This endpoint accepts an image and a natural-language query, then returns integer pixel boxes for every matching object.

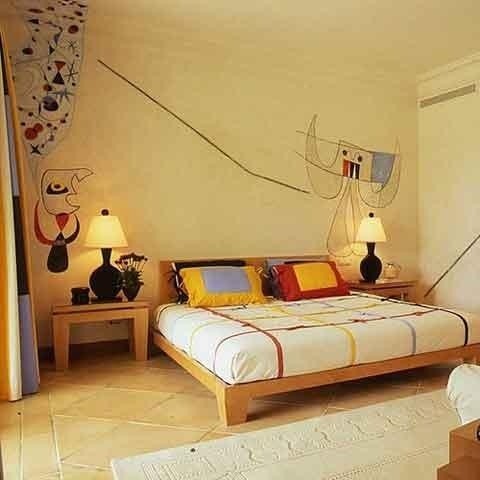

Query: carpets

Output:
[107,388,461,480]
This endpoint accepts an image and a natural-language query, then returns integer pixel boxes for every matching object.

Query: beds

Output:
[152,251,480,427]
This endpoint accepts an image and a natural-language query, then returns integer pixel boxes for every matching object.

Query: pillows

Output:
[179,266,270,309]
[267,261,352,303]
[265,258,313,300]
[169,258,247,305]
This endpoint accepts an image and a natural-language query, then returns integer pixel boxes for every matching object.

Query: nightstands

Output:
[350,277,419,305]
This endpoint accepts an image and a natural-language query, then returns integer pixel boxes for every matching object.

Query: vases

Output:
[121,283,141,302]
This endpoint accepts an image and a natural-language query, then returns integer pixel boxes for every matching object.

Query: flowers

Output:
[113,251,150,287]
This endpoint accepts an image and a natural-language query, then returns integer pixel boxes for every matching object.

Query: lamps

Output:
[354,210,388,284]
[84,207,129,300]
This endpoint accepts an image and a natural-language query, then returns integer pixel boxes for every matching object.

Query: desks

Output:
[49,294,154,373]
